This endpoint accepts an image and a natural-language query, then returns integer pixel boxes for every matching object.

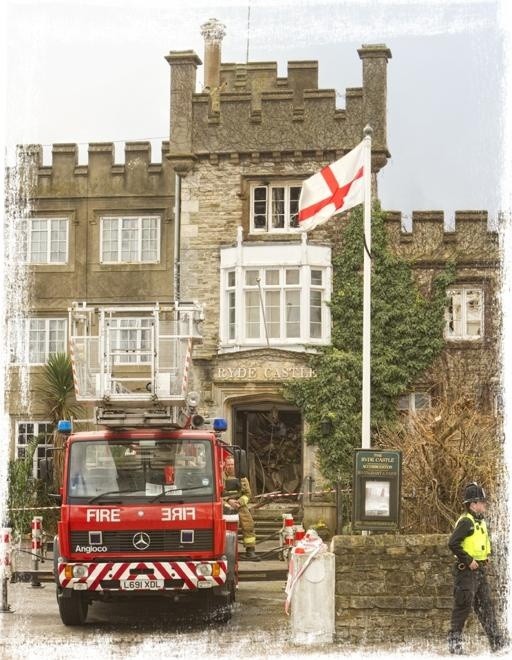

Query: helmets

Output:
[461,484,487,505]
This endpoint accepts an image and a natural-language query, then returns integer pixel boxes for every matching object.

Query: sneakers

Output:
[446,630,511,655]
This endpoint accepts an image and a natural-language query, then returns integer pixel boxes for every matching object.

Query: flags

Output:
[297,137,364,233]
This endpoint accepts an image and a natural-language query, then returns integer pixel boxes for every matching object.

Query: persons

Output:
[222,455,261,563]
[446,481,511,656]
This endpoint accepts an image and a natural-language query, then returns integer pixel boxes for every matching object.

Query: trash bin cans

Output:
[291,552,335,643]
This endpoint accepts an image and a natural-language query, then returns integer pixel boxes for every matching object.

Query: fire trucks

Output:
[44,412,248,626]
